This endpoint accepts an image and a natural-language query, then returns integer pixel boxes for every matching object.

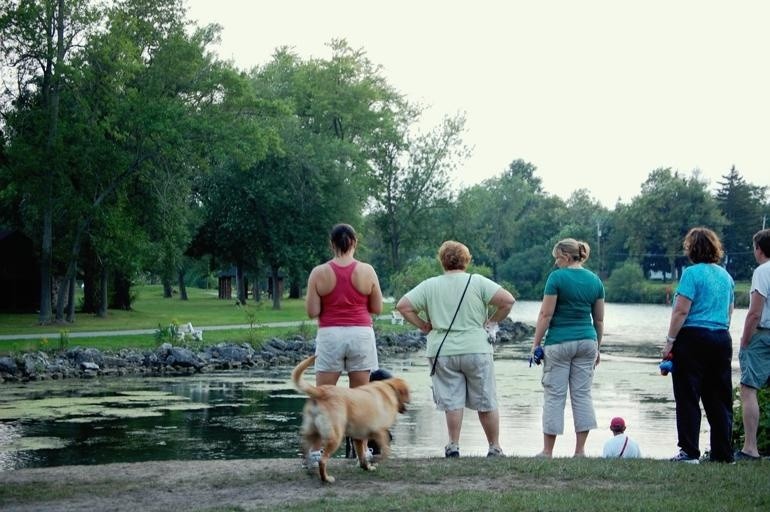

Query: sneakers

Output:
[670,450,700,465]
[701,455,736,465]
[487,446,504,457]
[356,448,374,468]
[301,449,321,468]
[444,442,460,457]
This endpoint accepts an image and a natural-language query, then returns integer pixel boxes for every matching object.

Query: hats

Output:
[610,417,625,430]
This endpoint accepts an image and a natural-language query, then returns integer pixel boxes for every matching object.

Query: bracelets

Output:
[665,334,677,346]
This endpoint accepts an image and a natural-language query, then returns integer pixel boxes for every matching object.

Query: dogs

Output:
[290,354,411,484]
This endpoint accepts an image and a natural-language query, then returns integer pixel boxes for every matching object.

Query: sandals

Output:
[734,451,761,464]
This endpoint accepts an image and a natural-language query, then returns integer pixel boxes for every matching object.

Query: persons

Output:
[529,237,599,457]
[603,417,640,455]
[732,229,769,461]
[303,223,383,470]
[658,226,735,465]
[394,239,515,458]
